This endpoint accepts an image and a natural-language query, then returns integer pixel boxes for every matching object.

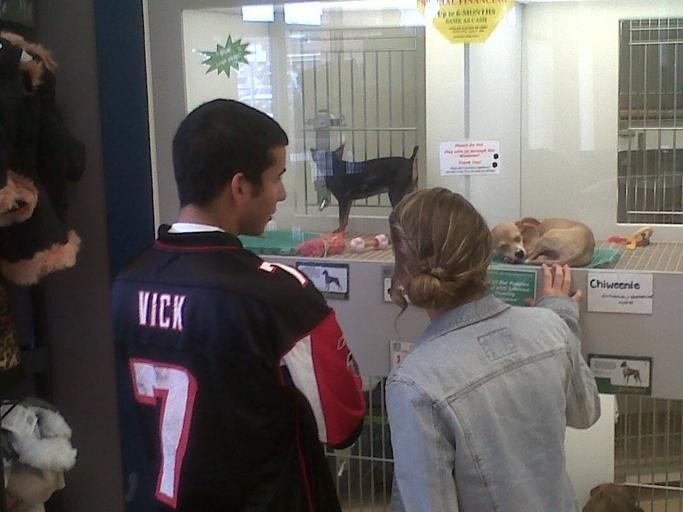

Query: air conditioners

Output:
[620,361,643,384]
[490,217,596,268]
[310,142,419,234]
[322,270,341,292]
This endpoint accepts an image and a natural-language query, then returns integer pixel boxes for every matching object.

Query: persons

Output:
[109,99,365,511]
[382,187,599,511]
